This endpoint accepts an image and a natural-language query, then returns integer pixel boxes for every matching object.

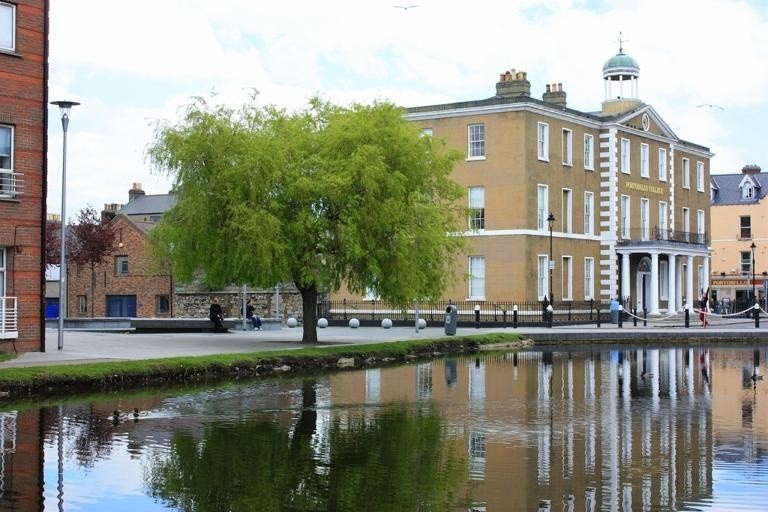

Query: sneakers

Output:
[254,326,264,330]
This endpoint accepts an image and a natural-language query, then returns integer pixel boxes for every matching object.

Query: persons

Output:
[241,299,264,331]
[209,296,224,327]
[700,284,710,326]
[700,351,713,387]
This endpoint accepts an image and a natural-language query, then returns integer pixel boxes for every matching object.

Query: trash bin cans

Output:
[445,305,456,335]
[610,300,619,323]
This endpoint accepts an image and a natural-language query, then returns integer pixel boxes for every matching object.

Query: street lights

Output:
[545,212,554,305]
[50,99,80,351]
[749,242,756,319]
